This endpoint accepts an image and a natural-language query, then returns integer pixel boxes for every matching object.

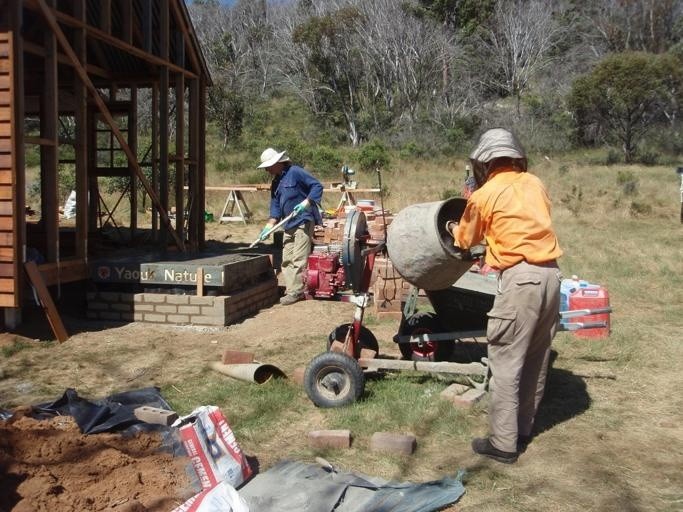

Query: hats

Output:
[256,148,287,169]
[468,127,524,163]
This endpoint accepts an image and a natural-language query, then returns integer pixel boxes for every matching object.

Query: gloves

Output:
[293,199,311,214]
[259,223,274,241]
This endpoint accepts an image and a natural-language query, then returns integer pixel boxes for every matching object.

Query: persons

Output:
[449,127,564,462]
[257,147,324,305]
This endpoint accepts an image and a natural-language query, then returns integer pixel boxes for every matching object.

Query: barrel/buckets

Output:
[569,287,613,340]
[569,282,601,295]
[560,274,586,330]
[385,196,480,291]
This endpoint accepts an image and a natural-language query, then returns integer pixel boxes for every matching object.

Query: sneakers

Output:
[471,437,518,464]
[280,292,306,304]
[518,435,529,453]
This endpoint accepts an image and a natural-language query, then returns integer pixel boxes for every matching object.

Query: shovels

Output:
[227,211,298,252]
